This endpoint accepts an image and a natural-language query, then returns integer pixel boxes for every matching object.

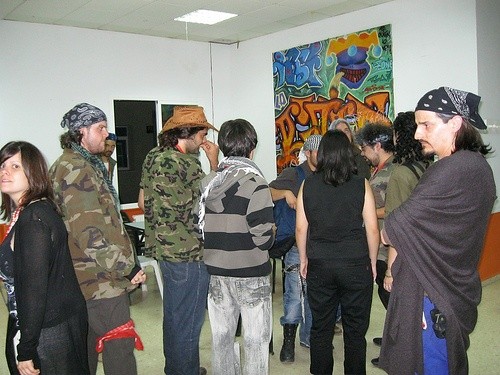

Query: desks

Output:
[125,222,146,255]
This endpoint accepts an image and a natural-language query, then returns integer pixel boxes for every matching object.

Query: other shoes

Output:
[164,366,207,375]
[371,358,380,366]
[373,338,382,345]
[334,326,342,333]
[300,342,334,350]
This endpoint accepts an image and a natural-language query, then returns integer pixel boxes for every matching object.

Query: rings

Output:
[135,278,139,282]
[141,272,145,276]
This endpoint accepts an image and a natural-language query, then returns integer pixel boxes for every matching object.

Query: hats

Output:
[160,104,220,133]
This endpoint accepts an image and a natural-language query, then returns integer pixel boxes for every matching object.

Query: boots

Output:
[280,324,299,363]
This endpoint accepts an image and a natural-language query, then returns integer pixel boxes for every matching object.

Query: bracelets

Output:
[385,274,392,277]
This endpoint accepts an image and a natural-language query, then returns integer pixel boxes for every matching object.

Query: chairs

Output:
[137,255,164,300]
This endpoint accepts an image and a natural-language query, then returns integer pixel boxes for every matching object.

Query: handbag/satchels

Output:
[268,235,295,258]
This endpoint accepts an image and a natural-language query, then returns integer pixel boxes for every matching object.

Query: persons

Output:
[381,87,498,375]
[295,129,380,375]
[0,142,89,375]
[138,106,220,375]
[356,112,435,369]
[268,135,323,364]
[47,103,146,375]
[203,119,277,374]
[328,119,361,335]
